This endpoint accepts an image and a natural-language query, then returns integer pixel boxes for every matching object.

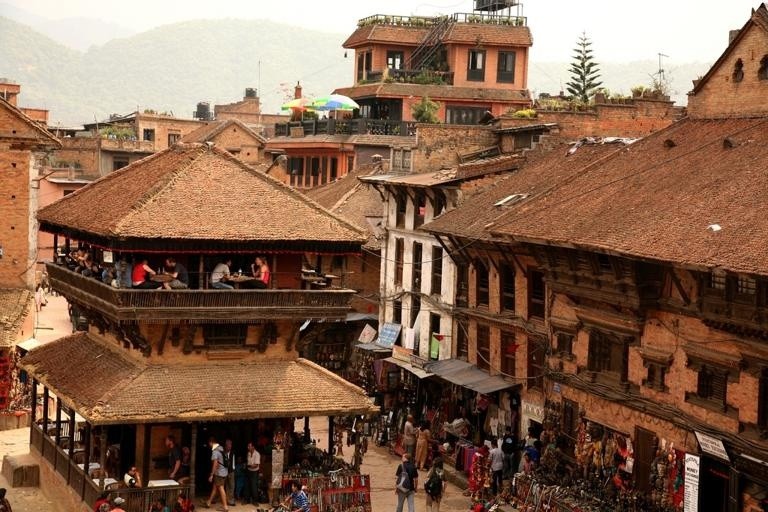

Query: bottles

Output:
[238,267,242,277]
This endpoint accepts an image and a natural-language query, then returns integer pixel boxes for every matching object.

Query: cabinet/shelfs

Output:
[513,473,599,511]
[315,343,346,376]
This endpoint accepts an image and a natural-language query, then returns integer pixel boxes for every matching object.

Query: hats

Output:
[114,497,126,504]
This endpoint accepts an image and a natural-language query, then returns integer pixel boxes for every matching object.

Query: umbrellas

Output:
[312,94,361,119]
[281,99,313,121]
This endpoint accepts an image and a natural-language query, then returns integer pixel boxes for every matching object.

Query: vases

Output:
[274,442,283,452]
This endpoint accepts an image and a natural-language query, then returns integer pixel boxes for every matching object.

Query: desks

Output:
[395,432,443,471]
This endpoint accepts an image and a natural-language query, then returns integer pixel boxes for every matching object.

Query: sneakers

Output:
[202,498,260,511]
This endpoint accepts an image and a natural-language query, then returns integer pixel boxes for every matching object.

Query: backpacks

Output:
[424,466,442,503]
[214,448,229,468]
[395,462,410,494]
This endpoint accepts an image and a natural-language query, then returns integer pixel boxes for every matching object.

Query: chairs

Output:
[64,247,342,290]
[37,417,189,509]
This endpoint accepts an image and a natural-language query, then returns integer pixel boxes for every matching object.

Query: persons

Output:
[65,248,133,289]
[36,283,45,312]
[163,258,190,290]
[68,305,81,333]
[0,488,12,512]
[210,258,234,289]
[245,257,271,290]
[131,257,157,289]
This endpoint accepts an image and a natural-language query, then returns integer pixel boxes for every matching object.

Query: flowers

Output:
[270,427,292,447]
